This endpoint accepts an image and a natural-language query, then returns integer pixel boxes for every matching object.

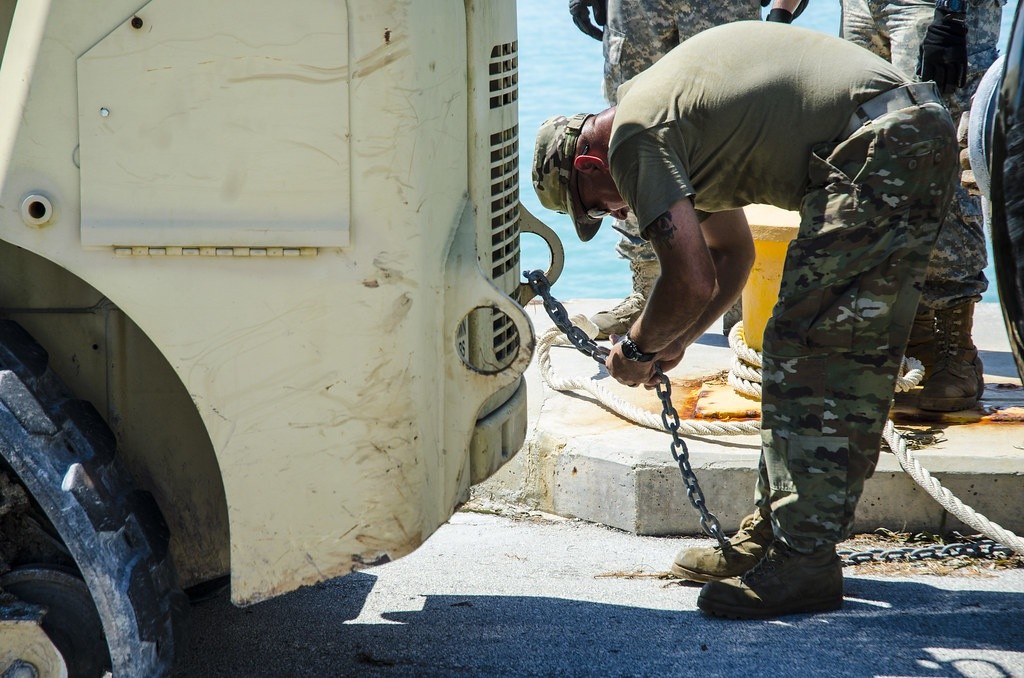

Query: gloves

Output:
[914,0,970,96]
[568,0,606,41]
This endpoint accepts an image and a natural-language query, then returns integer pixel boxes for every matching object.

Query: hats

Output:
[533,113,603,242]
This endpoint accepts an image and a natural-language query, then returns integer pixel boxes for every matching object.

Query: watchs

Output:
[620,330,658,363]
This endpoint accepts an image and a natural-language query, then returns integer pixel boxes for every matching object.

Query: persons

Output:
[531,20,960,620]
[569,0,803,340]
[839,0,1007,422]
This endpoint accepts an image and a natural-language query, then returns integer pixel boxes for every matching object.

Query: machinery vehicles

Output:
[0,0,565,678]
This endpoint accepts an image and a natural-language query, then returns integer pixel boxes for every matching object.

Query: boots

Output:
[904,300,985,410]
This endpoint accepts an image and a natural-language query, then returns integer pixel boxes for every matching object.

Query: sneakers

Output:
[672,508,774,585]
[697,537,844,619]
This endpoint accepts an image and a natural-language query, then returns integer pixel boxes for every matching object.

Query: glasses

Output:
[575,143,611,221]
